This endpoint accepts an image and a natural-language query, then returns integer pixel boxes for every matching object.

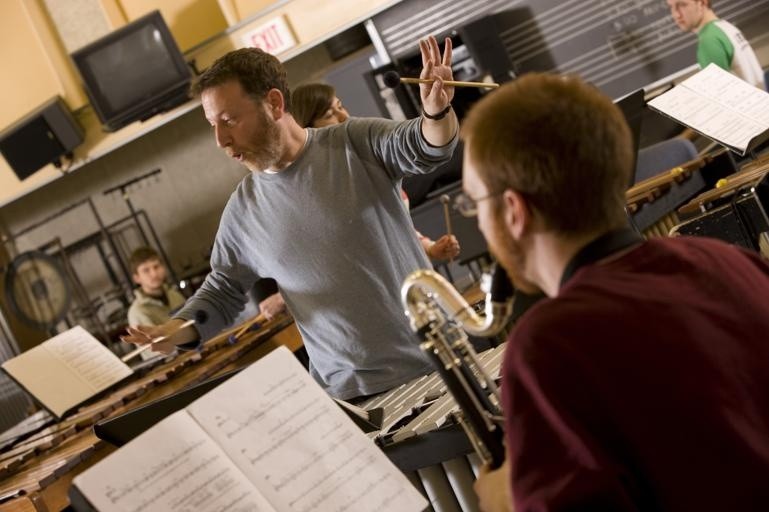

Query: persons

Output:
[119,37,460,405]
[259,83,454,326]
[127,247,184,365]
[461,75,769,512]
[664,0,766,96]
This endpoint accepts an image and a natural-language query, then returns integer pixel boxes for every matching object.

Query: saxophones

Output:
[399,260,515,471]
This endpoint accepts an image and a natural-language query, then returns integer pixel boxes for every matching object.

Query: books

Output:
[6,325,133,419]
[332,398,370,419]
[645,64,769,152]
[71,344,428,511]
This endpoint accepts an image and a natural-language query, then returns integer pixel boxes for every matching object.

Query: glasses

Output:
[451,190,509,218]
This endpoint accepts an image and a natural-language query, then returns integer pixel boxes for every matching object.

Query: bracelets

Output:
[423,106,450,121]
[427,242,435,258]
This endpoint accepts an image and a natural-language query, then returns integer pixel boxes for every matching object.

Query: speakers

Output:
[0,97,88,181]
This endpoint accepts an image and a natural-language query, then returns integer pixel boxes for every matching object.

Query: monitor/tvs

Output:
[69,9,204,133]
